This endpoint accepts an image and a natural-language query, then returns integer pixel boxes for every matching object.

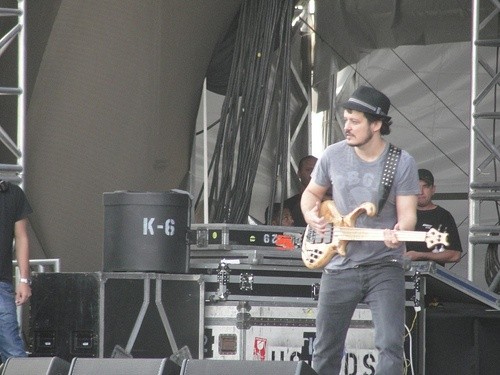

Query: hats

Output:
[341,86,391,121]
[418,169,434,186]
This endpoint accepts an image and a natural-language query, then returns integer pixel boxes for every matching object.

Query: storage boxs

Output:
[27,269,205,364]
[191,223,307,253]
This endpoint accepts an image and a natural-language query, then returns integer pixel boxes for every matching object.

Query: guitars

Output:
[300,198,452,269]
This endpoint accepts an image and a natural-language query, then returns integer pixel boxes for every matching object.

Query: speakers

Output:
[68,358,182,374]
[180,358,320,375]
[2,356,70,375]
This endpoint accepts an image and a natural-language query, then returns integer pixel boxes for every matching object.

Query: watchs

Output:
[19,278,33,287]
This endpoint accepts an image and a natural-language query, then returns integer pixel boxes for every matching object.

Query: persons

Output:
[265,204,296,228]
[300,86,423,375]
[0,176,34,360]
[399,168,463,267]
[286,155,320,226]
